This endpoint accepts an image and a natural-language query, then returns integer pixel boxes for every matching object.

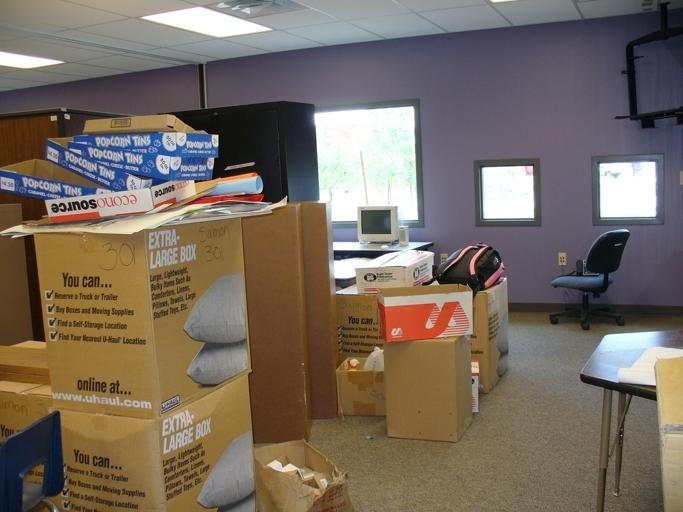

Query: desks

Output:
[579,328,683,512]
[334,241,434,258]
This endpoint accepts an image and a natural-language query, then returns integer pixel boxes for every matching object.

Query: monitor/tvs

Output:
[356,206,398,242]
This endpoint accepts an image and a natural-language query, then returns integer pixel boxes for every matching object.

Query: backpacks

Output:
[421,243,506,298]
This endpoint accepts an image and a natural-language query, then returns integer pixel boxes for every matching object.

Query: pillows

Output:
[187,341,247,385]
[197,428,255,509]
[184,275,247,343]
[217,493,254,512]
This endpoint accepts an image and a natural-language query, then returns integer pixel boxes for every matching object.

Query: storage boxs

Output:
[48,374,256,512]
[376,282,474,343]
[384,335,473,443]
[471,277,510,394]
[253,439,355,512]
[335,284,385,363]
[300,201,340,419]
[33,216,252,417]
[355,250,434,294]
[336,356,385,417]
[243,204,310,445]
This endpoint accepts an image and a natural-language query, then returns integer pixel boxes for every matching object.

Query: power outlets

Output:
[557,253,567,266]
[440,253,448,264]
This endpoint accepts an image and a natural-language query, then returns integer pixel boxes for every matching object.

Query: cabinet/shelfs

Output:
[156,100,320,202]
[0,108,134,341]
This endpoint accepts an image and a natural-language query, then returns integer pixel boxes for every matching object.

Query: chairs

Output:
[0,410,64,512]
[547,229,631,330]
